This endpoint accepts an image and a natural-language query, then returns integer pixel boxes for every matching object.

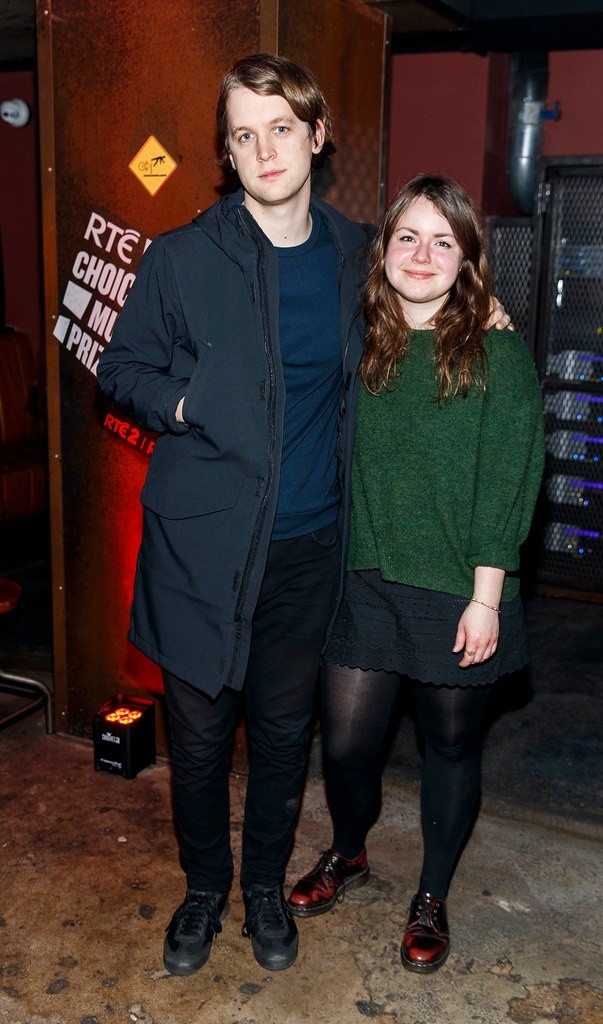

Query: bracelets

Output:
[469,597,500,613]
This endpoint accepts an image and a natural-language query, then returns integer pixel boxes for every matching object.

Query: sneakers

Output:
[400,886,452,975]
[242,879,300,971]
[287,845,372,916]
[162,882,231,976]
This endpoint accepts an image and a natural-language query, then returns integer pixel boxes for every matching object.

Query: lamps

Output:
[92,699,154,780]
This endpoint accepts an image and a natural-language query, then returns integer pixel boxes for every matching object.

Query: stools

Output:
[0,580,54,736]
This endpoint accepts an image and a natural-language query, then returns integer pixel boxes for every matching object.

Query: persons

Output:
[95,54,518,976]
[281,171,547,976]
